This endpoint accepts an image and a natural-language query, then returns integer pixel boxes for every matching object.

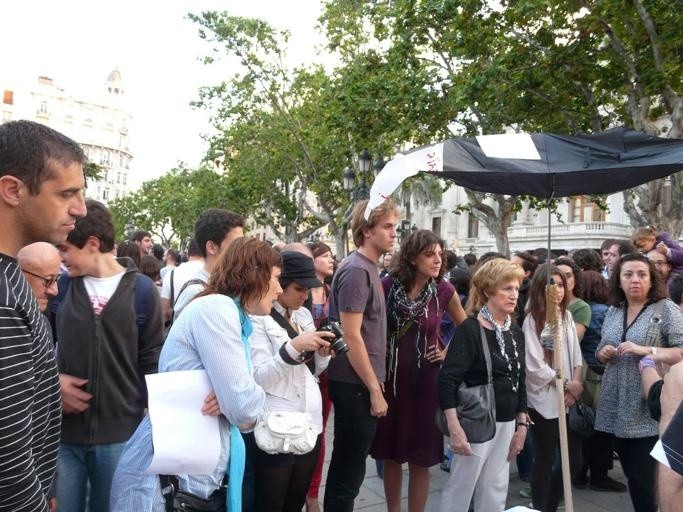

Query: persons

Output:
[14,198,683,511]
[0,121,87,509]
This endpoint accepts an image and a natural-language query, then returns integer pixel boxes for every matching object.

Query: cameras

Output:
[318,319,349,360]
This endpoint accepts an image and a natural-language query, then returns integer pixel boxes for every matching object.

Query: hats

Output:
[280,252,324,288]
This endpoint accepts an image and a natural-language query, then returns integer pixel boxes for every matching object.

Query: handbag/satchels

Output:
[254,411,318,455]
[435,382,496,443]
[569,400,594,446]
[165,488,227,512]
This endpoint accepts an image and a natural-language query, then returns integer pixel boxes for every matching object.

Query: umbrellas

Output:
[366,127,683,337]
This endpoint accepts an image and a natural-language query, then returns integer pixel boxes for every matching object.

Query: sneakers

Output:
[520,485,565,509]
[589,476,627,492]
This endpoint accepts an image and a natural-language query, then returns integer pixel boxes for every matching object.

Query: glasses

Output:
[22,269,60,288]
